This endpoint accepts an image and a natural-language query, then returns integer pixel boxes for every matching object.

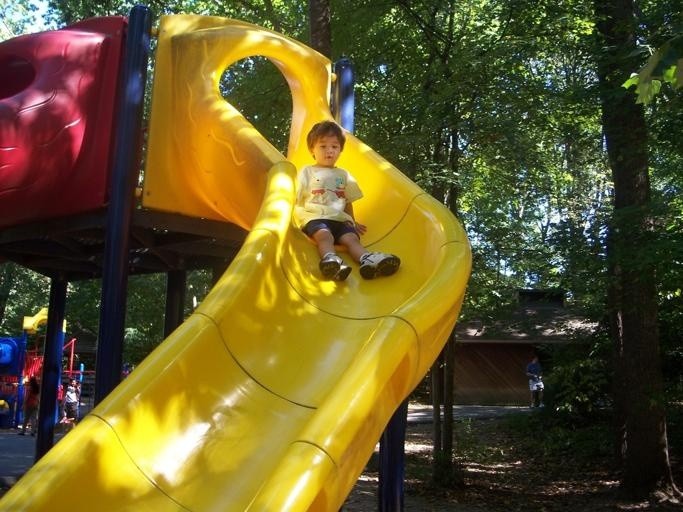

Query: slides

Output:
[0,14,471,511]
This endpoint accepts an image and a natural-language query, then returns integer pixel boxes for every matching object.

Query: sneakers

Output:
[359,253,400,279]
[319,254,352,281]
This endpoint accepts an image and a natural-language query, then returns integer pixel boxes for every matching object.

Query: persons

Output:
[16,375,38,437]
[54,379,84,434]
[292,119,402,284]
[524,353,545,410]
